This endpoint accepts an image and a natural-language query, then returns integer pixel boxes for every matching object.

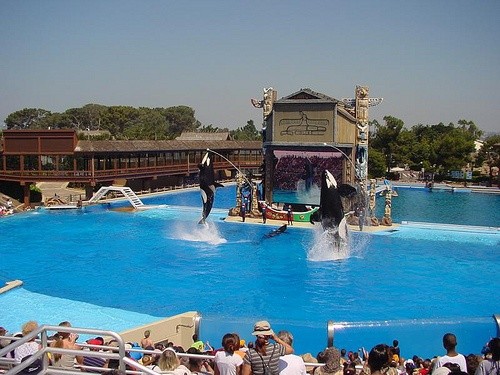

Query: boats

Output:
[259,199,320,222]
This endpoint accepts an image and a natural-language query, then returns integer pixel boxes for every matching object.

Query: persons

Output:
[288,205,293,225]
[241,203,246,222]
[261,202,267,224]
[0,199,13,215]
[1,320,500,375]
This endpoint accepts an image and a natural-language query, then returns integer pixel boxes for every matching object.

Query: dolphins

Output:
[254,224,289,244]
[197,153,224,225]
[309,169,357,258]
